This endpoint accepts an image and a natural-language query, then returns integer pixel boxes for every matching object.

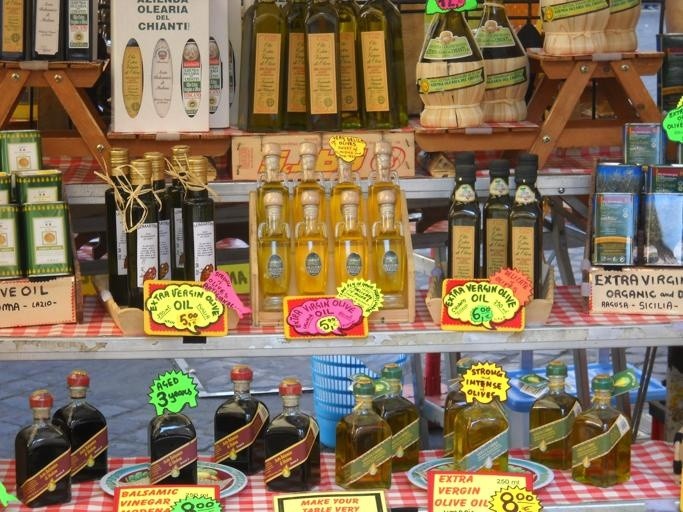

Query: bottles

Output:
[0,0,93,62]
[15,392,72,506]
[413,0,640,132]
[254,137,408,311]
[336,375,391,491]
[373,365,420,473]
[239,0,408,132]
[445,358,508,478]
[214,365,268,475]
[148,381,199,489]
[445,151,541,297]
[266,379,322,492]
[103,143,216,310]
[529,360,584,472]
[53,372,109,483]
[571,374,630,484]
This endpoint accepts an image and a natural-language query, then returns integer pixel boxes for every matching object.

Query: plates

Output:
[409,455,554,492]
[99,459,245,505]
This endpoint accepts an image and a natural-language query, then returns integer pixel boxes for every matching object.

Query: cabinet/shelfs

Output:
[0,136,683,511]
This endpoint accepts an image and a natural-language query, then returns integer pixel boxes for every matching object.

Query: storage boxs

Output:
[498,357,669,459]
